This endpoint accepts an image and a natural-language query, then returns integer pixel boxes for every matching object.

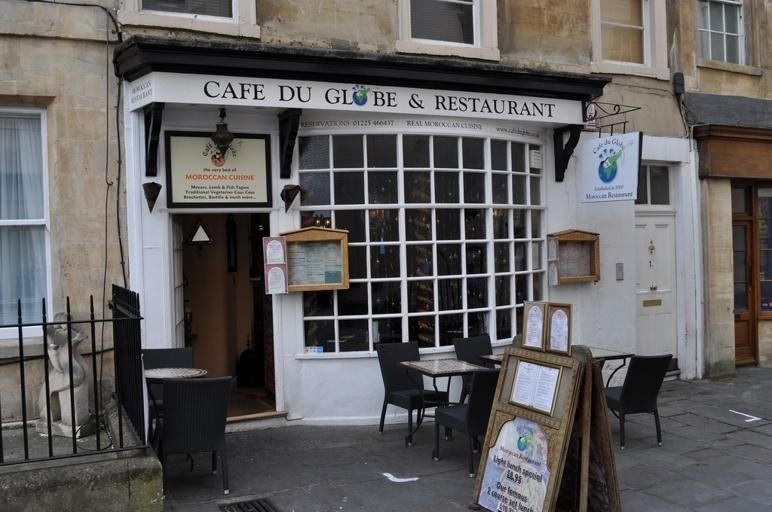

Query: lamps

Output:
[140,181,162,213]
[281,184,302,215]
[211,108,234,159]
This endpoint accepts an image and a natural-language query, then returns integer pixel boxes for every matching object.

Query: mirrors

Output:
[186,219,218,247]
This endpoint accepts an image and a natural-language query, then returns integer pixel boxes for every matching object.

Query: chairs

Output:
[141,346,234,496]
[605,353,674,450]
[373,333,502,480]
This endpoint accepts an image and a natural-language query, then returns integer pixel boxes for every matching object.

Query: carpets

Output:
[223,384,274,418]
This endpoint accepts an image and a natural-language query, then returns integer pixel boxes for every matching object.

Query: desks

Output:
[477,350,634,422]
